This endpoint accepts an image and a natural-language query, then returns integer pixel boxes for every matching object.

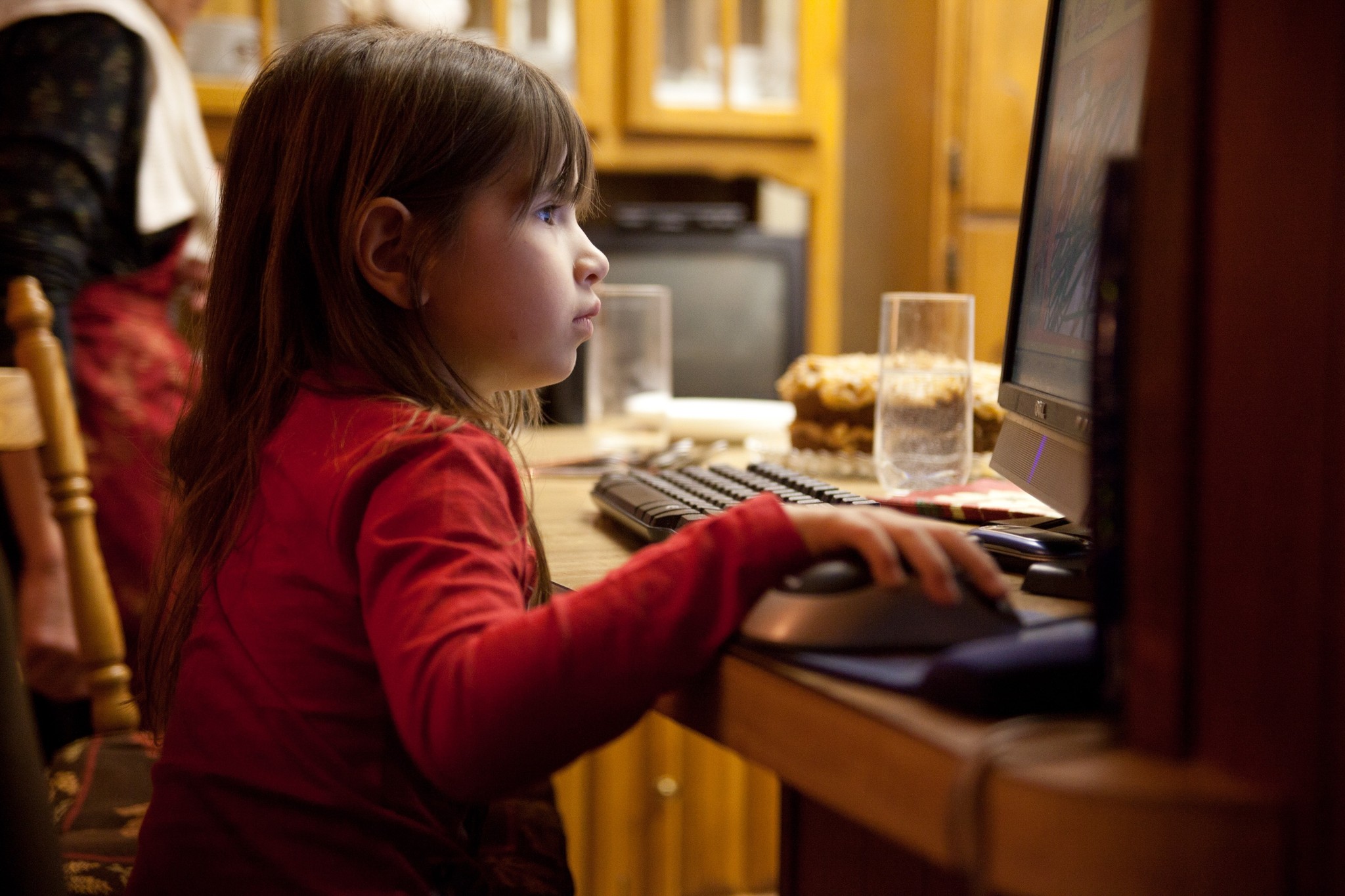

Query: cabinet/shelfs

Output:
[146,0,1048,895]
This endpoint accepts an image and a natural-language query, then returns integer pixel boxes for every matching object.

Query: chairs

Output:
[0,275,153,896]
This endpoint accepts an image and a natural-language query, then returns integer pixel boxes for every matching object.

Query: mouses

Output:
[741,550,1031,645]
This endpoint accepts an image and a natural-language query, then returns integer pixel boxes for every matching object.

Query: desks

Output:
[514,420,1283,896]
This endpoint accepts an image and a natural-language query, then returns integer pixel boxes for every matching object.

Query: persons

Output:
[126,22,1010,896]
[0,1,219,772]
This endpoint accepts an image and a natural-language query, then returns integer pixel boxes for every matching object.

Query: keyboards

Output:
[588,454,885,586]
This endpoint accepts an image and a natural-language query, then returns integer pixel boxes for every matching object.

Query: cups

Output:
[581,282,673,453]
[871,289,974,499]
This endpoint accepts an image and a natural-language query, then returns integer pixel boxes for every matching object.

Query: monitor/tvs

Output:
[984,0,1160,521]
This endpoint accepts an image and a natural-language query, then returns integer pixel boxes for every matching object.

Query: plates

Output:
[624,400,797,442]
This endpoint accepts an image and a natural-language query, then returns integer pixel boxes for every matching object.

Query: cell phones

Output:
[973,520,1083,576]
